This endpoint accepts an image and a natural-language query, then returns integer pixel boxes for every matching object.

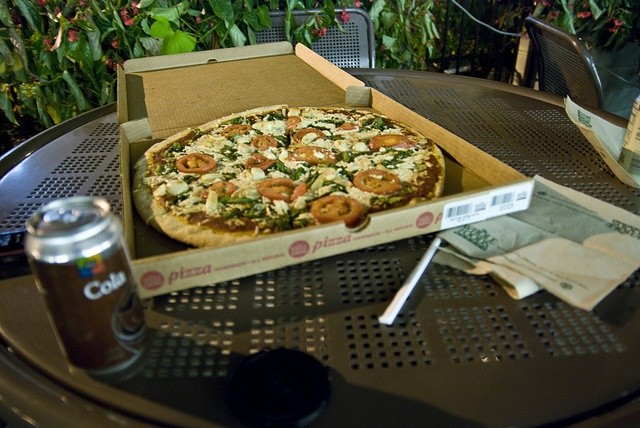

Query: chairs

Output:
[525,16,637,111]
[238,7,376,68]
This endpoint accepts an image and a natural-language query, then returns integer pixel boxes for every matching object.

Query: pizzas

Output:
[131,101,448,249]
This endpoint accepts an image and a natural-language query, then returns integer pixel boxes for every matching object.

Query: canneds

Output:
[23,196,159,377]
[22,290,24,293]
